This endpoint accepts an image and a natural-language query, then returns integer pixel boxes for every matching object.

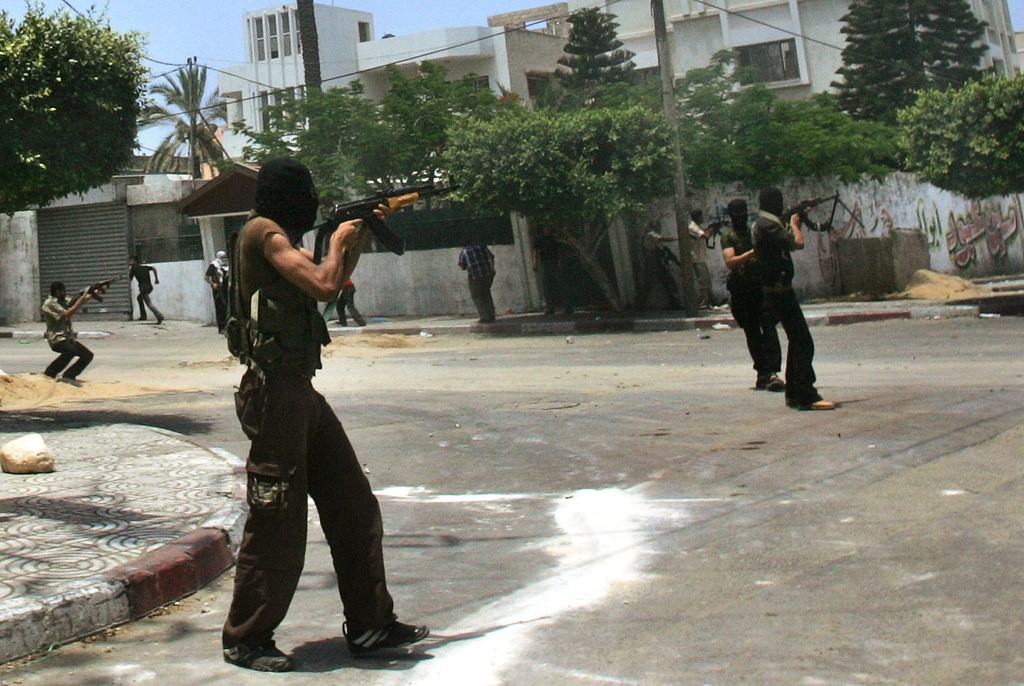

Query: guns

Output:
[779,189,865,233]
[308,173,462,266]
[663,244,680,266]
[78,274,127,302]
[701,220,730,249]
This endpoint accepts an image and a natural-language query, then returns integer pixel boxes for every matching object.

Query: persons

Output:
[631,219,682,305]
[40,282,108,383]
[687,209,729,309]
[205,250,231,335]
[333,280,368,325]
[457,233,497,324]
[533,224,576,315]
[216,157,434,678]
[752,189,845,411]
[721,198,784,392]
[128,257,164,324]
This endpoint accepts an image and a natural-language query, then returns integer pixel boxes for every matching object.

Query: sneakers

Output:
[800,396,835,411]
[344,620,428,654]
[768,374,783,391]
[222,637,297,672]
[756,373,770,386]
